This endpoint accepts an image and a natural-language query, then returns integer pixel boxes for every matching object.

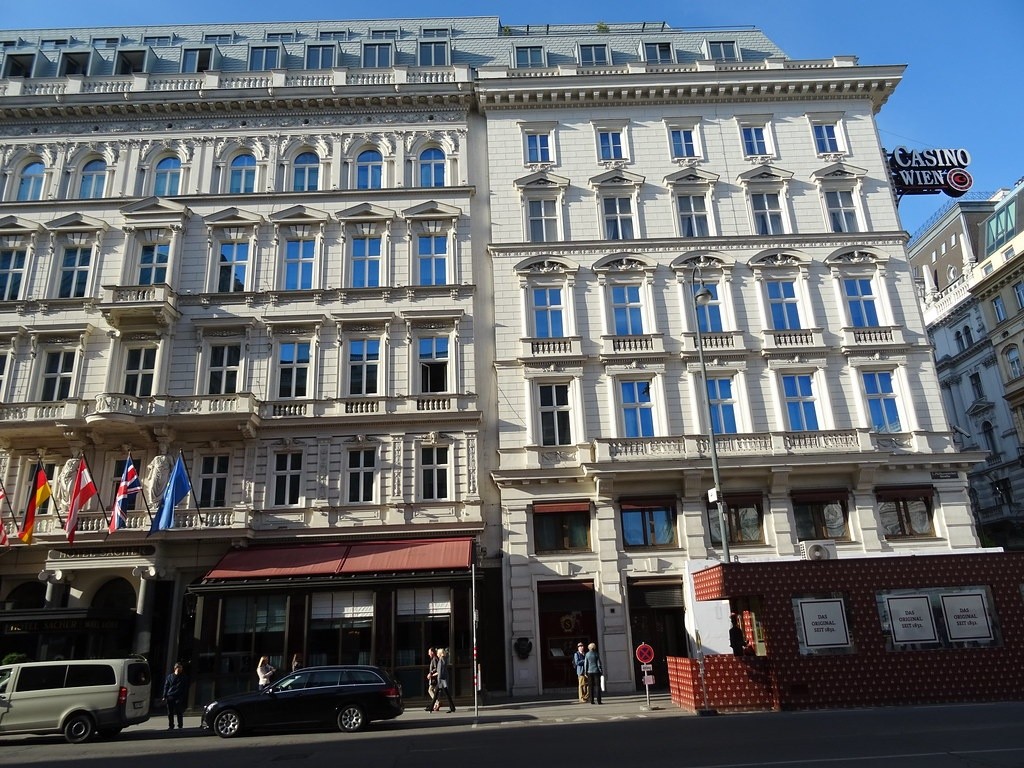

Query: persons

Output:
[162,662,184,731]
[574,643,590,703]
[427,648,441,711]
[424,648,456,713]
[257,656,276,690]
[292,653,303,672]
[729,615,747,656]
[584,643,603,705]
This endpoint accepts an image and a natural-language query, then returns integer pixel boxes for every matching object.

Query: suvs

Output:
[200,665,407,739]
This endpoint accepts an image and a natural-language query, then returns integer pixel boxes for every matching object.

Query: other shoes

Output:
[179,727,183,732]
[433,705,440,711]
[167,728,173,731]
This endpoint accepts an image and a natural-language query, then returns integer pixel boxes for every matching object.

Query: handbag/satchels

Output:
[601,676,605,691]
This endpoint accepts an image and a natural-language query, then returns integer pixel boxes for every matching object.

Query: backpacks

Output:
[572,652,579,669]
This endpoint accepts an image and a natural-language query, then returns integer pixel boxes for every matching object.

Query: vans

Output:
[0,653,152,746]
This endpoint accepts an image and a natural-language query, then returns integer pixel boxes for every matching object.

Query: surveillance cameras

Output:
[953,425,971,438]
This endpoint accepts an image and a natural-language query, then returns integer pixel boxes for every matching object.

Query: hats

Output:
[577,643,583,646]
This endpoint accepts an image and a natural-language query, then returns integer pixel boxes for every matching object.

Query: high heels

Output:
[446,709,455,713]
[424,708,432,713]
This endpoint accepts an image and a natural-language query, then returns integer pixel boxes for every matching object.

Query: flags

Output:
[145,454,192,539]
[108,454,143,535]
[0,481,9,547]
[63,454,97,544]
[18,456,53,545]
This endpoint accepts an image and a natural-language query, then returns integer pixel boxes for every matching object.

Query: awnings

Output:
[203,536,472,579]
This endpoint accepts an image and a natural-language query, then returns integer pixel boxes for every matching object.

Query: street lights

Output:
[691,261,731,567]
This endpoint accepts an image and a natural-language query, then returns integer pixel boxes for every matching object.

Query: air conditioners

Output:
[799,539,837,559]
